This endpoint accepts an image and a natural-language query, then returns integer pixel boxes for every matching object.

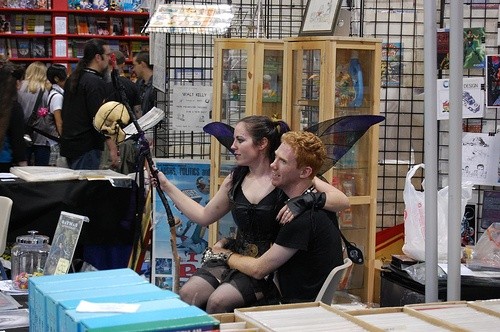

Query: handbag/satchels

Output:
[403,164,471,263]
[29,93,61,142]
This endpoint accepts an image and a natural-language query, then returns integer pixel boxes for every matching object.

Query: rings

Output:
[286,211,289,213]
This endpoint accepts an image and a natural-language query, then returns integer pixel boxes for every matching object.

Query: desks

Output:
[0,178,145,273]
[380,269,447,307]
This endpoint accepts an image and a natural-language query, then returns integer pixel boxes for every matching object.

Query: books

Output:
[0,0,154,85]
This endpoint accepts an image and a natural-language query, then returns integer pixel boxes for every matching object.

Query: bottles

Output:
[348,49,364,108]
[12,230,51,289]
[332,172,356,226]
[263,60,279,102]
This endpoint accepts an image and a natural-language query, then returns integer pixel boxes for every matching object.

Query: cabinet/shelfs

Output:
[0,0,150,73]
[208,37,382,303]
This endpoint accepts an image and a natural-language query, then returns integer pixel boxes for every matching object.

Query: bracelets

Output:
[222,250,234,262]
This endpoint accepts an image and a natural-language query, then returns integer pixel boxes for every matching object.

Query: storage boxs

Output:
[29,267,497,332]
[390,255,422,270]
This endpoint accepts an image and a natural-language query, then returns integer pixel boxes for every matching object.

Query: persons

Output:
[145,115,351,315]
[1,39,155,174]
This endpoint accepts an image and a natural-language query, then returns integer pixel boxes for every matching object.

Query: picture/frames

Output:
[298,0,343,36]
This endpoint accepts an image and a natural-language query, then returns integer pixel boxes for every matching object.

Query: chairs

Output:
[314,257,353,305]
